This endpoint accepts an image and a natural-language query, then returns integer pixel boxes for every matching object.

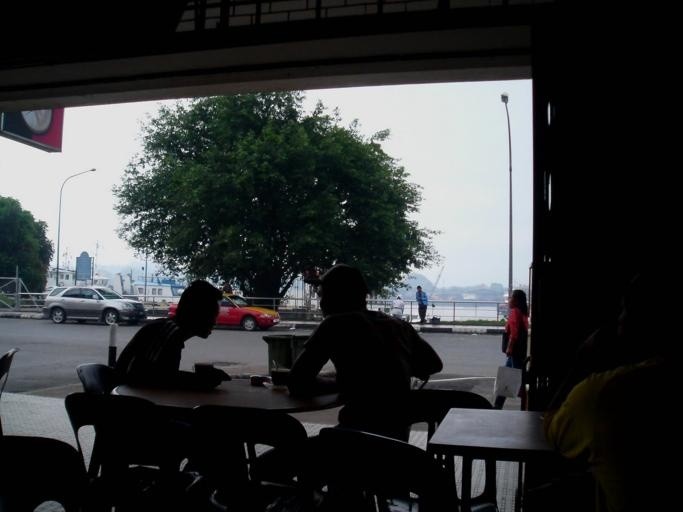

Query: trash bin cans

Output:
[262,331,312,388]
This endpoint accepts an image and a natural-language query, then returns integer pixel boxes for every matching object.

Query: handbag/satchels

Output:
[493,354,523,399]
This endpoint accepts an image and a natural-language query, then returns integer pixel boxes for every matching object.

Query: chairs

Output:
[319,389,496,512]
[0,348,117,512]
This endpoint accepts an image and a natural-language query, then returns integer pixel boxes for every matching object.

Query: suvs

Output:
[44,287,148,324]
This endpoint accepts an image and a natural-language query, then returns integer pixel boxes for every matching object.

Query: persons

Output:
[108,282,222,460]
[392,297,404,318]
[416,286,428,324]
[493,290,529,410]
[251,260,444,485]
[222,283,232,294]
[547,317,683,512]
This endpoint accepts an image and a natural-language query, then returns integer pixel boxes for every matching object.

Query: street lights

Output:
[498,91,514,298]
[56,167,96,288]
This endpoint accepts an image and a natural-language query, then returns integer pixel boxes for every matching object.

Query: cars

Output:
[168,294,281,331]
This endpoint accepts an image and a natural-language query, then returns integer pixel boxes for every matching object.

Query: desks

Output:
[110,378,346,512]
[428,408,563,512]
